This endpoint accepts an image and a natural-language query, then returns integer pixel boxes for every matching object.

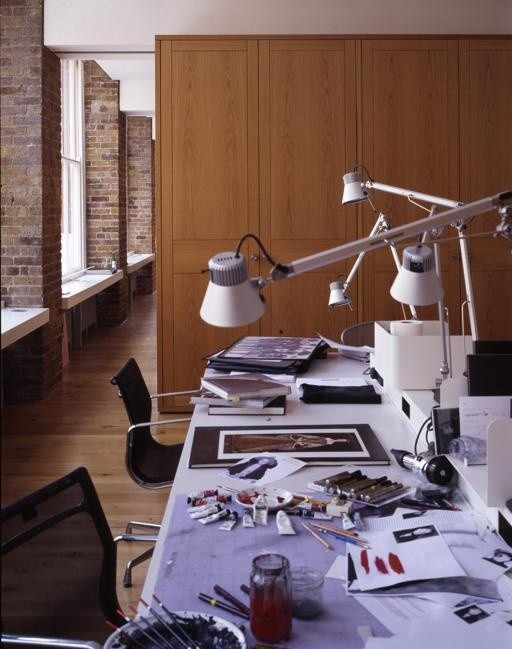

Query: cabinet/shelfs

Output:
[356,34,510,355]
[154,33,357,413]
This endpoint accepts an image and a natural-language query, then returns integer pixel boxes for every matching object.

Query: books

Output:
[187,370,290,415]
[188,423,392,469]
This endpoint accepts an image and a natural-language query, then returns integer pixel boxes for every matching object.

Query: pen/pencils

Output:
[197,584,253,621]
[301,521,371,549]
[313,470,411,505]
[218,484,238,493]
[11,309,27,311]
[269,495,332,518]
[401,497,461,511]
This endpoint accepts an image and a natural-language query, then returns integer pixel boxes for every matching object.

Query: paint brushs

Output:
[106,595,200,649]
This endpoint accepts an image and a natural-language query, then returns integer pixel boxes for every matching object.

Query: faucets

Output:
[68,271,87,281]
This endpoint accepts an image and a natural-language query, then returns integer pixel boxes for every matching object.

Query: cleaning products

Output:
[111,252,118,271]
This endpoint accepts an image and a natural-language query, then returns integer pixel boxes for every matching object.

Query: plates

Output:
[101,609,246,649]
[234,486,294,513]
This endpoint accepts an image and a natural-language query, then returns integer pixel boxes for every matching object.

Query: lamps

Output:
[338,163,480,342]
[389,204,450,382]
[199,191,511,331]
[326,212,421,321]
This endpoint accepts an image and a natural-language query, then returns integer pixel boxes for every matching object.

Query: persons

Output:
[223,434,350,452]
[226,455,277,485]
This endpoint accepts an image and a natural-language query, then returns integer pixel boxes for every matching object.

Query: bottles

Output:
[111,251,117,272]
[248,553,294,640]
[447,435,487,465]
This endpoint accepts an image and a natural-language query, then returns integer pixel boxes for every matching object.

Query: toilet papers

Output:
[390,320,424,336]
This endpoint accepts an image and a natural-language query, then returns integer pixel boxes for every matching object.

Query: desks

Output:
[136,343,512,648]
[1,302,52,351]
[62,269,125,351]
[127,248,155,304]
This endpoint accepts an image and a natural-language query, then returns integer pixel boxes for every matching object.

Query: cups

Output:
[288,565,325,619]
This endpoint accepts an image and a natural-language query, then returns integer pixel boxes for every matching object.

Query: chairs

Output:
[113,358,202,590]
[1,468,135,648]
